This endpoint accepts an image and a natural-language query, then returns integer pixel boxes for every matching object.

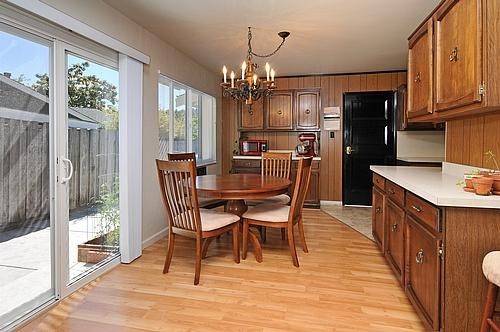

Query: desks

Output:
[179,174,292,264]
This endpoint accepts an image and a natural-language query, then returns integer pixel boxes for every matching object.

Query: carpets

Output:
[320,201,375,242]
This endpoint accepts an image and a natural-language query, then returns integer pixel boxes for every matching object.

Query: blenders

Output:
[295,133,317,157]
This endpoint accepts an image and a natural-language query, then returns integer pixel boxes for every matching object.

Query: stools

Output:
[479,251,500,332]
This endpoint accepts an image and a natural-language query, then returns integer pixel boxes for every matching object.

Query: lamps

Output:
[219,27,278,106]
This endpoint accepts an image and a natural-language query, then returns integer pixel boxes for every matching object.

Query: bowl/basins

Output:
[297,145,308,154]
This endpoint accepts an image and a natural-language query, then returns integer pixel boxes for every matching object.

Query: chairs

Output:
[241,156,313,268]
[155,158,241,285]
[261,151,293,240]
[168,152,229,211]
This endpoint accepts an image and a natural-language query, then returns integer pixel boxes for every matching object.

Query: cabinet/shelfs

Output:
[405,0,500,123]
[237,88,321,132]
[232,159,321,209]
[396,83,445,131]
[372,173,500,332]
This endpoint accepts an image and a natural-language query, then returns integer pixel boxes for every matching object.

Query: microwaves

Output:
[239,139,268,156]
